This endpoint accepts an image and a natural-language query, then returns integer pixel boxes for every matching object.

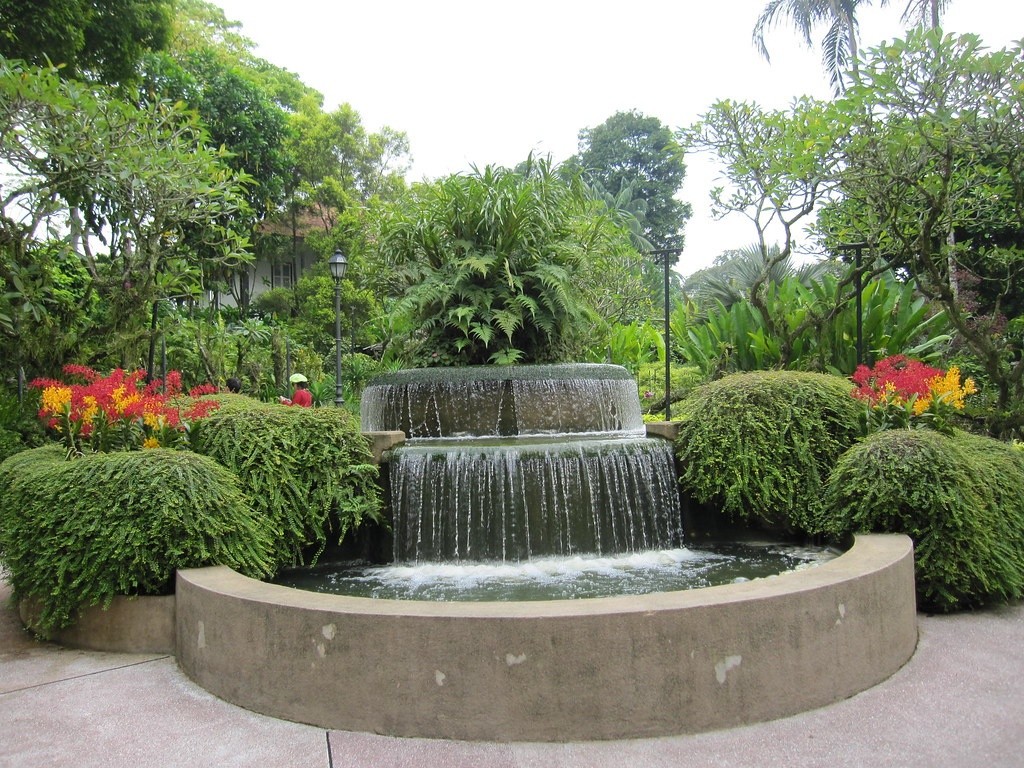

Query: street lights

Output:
[838,243,882,364]
[649,249,684,420]
[328,249,350,404]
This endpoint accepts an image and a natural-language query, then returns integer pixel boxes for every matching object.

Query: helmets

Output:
[226,378,241,393]
[289,373,308,382]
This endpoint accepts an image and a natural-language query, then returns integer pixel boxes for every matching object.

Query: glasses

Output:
[292,382,297,387]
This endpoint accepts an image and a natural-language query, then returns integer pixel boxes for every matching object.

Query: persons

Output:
[224,377,242,395]
[279,373,312,408]
